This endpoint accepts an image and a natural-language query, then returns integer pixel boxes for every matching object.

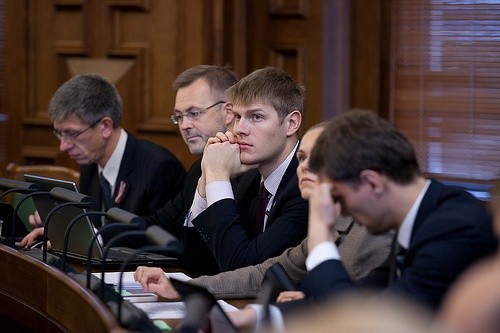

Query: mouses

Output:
[25,239,44,250]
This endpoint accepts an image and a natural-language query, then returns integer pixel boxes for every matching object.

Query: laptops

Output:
[169,277,238,333]
[23,174,176,264]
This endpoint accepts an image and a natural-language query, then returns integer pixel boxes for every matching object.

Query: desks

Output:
[0,244,271,332]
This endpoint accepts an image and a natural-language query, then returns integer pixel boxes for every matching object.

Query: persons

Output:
[133,119,400,306]
[176,65,314,272]
[20,65,242,271]
[21,72,191,257]
[218,106,500,333]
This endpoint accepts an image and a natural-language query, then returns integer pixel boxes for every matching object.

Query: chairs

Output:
[6,161,81,235]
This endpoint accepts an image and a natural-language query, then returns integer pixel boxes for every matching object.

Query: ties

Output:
[254,182,271,238]
[378,235,405,289]
[99,171,112,225]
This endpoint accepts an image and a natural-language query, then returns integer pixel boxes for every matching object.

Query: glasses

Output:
[171,101,226,125]
[53,115,115,143]
